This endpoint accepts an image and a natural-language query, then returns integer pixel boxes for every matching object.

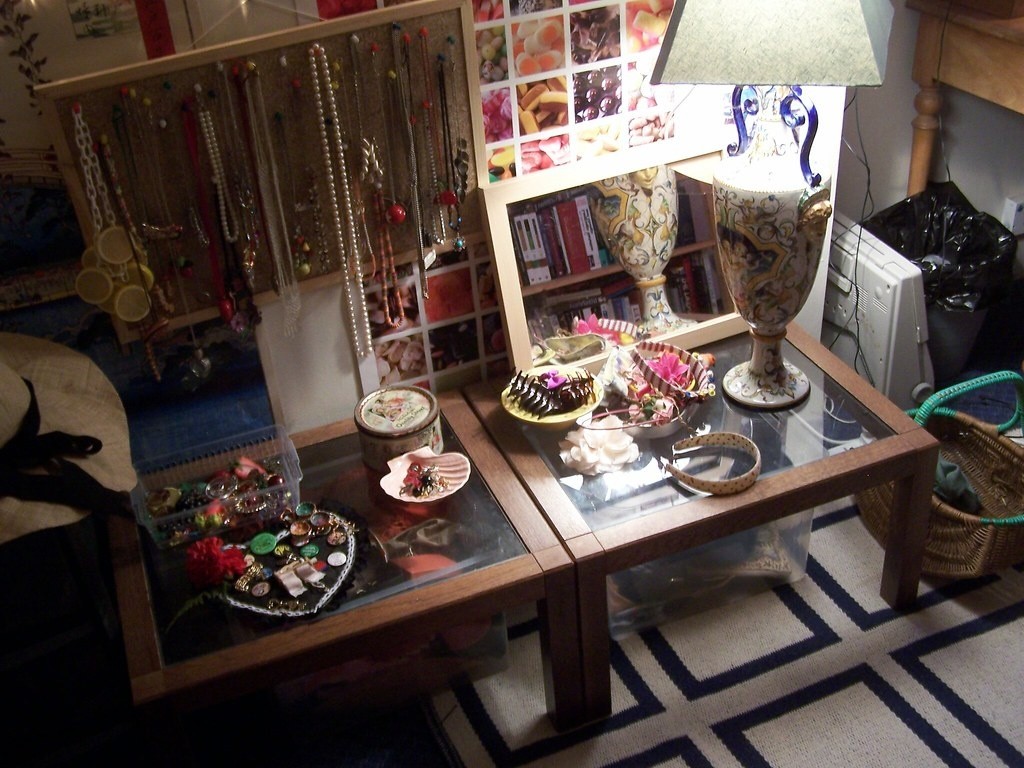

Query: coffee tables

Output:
[108,390,586,768]
[467,315,944,724]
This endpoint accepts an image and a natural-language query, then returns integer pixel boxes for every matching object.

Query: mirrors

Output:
[477,127,750,378]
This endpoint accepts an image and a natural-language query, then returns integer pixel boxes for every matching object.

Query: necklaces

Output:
[71,28,468,382]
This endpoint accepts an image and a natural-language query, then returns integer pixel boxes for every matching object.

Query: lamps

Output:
[649,0,881,410]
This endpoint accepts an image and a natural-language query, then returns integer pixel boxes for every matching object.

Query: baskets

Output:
[131,425,303,551]
[852,371,1024,580]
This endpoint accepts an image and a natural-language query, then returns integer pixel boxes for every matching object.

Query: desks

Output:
[905,0,1024,201]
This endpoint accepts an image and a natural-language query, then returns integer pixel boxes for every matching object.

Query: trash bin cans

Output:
[860,180,1017,385]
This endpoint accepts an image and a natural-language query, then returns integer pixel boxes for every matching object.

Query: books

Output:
[508,179,724,345]
